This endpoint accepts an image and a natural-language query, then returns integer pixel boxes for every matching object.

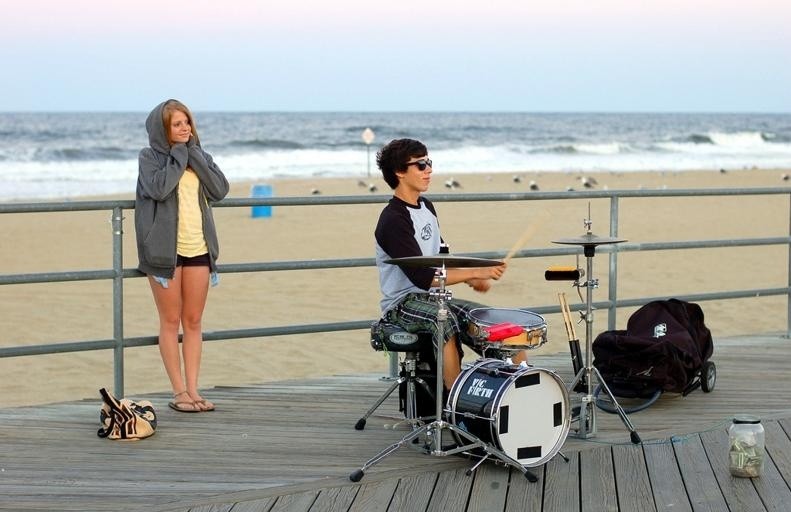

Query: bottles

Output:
[727,415,765,478]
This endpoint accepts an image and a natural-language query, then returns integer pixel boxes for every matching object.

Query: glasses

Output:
[406,159,432,170]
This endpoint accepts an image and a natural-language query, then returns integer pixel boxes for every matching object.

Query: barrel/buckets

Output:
[251,184,272,217]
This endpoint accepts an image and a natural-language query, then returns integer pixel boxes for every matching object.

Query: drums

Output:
[466,308,547,350]
[447,358,572,469]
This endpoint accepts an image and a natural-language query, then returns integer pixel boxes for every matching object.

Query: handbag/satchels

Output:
[97,387,157,442]
[592,297,711,400]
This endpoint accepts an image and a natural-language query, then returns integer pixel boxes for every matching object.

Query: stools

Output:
[355,323,436,430]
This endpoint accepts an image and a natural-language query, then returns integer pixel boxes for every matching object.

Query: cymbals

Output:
[553,235,629,245]
[383,257,505,267]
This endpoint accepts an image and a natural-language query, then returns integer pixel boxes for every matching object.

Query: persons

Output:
[135,98,229,412]
[374,139,529,408]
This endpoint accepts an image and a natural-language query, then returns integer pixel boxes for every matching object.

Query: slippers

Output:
[196,399,215,411]
[169,400,201,412]
